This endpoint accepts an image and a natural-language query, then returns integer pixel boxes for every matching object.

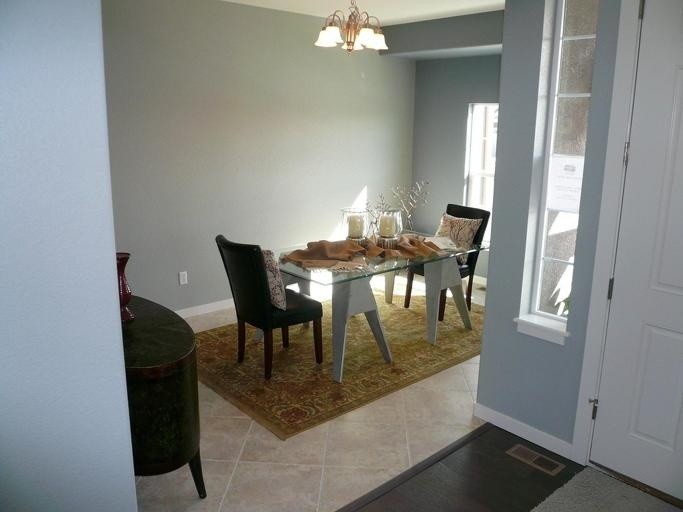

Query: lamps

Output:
[313,0,389,54]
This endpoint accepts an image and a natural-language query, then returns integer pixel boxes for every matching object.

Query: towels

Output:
[366,238,449,261]
[282,240,367,270]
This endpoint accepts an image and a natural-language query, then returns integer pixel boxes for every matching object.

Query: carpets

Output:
[194,286,485,440]
[335,422,683,512]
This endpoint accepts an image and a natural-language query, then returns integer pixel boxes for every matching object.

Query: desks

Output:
[121,295,208,498]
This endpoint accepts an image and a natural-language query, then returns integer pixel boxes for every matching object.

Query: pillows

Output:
[434,212,482,266]
[260,249,287,312]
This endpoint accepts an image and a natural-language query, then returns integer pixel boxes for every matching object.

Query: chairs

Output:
[216,234,323,379]
[405,204,490,321]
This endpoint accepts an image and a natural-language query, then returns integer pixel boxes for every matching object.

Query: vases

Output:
[115,252,136,320]
